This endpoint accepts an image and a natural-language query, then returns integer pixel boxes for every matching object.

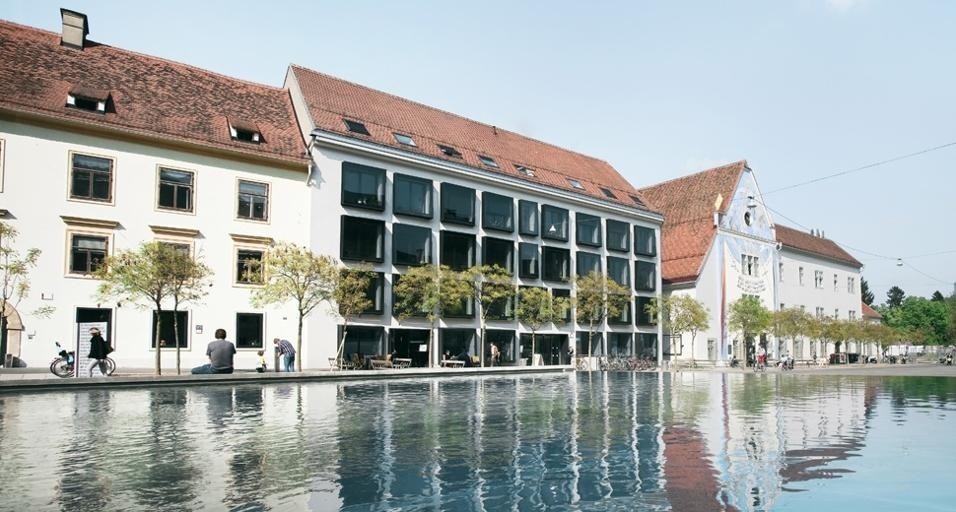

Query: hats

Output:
[88,328,100,334]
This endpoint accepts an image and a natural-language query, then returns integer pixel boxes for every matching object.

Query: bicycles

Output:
[752,355,766,372]
[50,349,116,378]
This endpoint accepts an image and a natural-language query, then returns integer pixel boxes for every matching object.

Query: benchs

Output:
[440,359,466,368]
[328,352,414,373]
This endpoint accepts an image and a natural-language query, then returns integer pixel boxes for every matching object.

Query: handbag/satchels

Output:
[101,337,112,355]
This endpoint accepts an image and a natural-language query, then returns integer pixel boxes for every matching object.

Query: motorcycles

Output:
[778,355,795,372]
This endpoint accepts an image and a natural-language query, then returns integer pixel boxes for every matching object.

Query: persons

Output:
[445,344,472,367]
[776,349,794,371]
[730,355,739,368]
[941,352,952,368]
[748,341,766,369]
[255,350,266,373]
[490,341,500,367]
[191,329,236,374]
[566,346,574,365]
[86,327,109,377]
[385,347,398,368]
[274,338,296,372]
